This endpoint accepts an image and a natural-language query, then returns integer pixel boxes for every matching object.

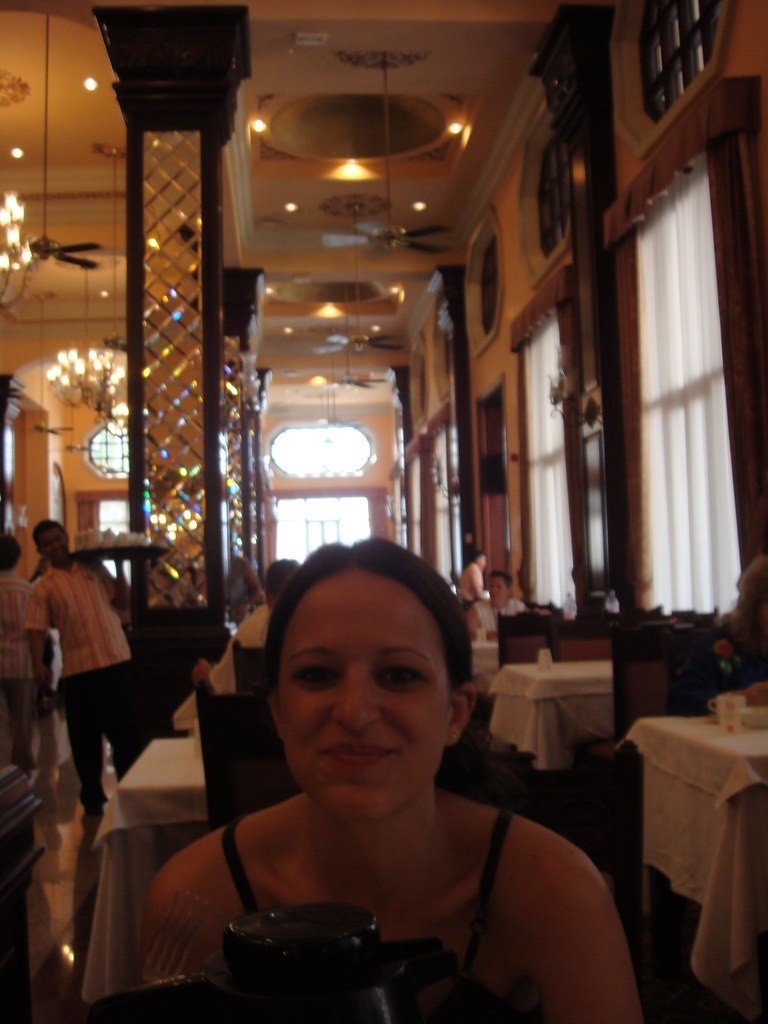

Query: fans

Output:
[315,275,406,354]
[320,344,389,389]
[28,10,103,271]
[318,52,465,253]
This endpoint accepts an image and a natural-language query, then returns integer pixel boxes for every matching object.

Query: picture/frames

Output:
[579,384,602,439]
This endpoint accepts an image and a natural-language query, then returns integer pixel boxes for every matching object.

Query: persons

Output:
[153,556,300,693]
[0,534,36,772]
[453,551,532,640]
[138,540,643,1024]
[666,558,768,716]
[21,519,145,824]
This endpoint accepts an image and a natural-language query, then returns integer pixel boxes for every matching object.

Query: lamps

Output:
[547,369,580,404]
[0,190,35,308]
[46,268,126,412]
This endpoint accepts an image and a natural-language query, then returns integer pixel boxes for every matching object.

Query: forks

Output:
[140,893,207,988]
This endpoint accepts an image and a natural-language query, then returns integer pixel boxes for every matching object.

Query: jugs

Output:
[83,898,458,1024]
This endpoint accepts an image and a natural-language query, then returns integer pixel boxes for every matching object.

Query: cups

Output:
[715,694,745,729]
[536,648,554,671]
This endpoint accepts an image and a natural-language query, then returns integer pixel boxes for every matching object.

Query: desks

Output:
[614,716,768,1022]
[488,661,615,773]
[80,736,211,1005]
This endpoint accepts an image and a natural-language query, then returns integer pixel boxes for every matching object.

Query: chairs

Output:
[553,610,614,661]
[611,617,700,744]
[232,637,269,691]
[497,611,562,667]
[194,678,299,835]
